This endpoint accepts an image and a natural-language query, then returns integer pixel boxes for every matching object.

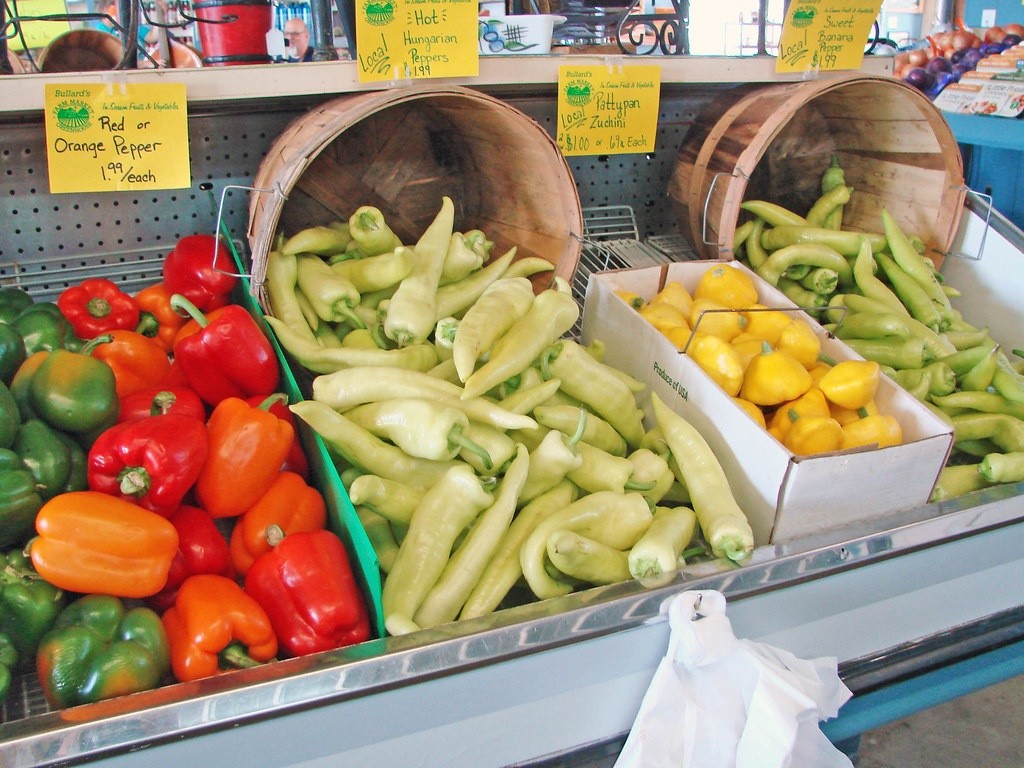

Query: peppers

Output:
[261,154,1024,640]
[0,233,370,728]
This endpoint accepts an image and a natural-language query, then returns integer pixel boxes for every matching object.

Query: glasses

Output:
[285,31,305,37]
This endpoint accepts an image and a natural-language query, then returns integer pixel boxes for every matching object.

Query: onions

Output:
[890,23,1024,79]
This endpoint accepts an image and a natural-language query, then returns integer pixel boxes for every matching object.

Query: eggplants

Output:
[906,34,1022,92]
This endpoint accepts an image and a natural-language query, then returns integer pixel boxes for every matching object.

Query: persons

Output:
[282,18,315,62]
[137,1,168,63]
[96,1,119,37]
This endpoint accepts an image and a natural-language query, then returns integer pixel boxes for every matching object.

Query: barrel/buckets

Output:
[39,28,122,74]
[192,0,271,65]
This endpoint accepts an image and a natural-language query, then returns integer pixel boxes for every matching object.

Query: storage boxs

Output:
[581,260,956,548]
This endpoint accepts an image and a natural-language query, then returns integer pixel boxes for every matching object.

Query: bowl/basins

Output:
[477,14,567,56]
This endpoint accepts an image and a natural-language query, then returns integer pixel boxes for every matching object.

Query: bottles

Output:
[139,1,194,48]
[272,1,312,32]
